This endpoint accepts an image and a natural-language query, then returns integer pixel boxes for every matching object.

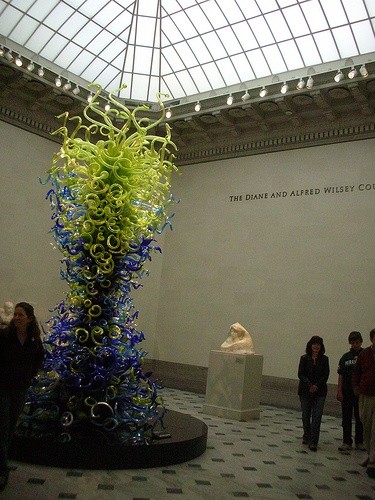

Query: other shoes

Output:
[0,475,8,491]
[309,442,317,452]
[367,460,375,478]
[356,442,363,451]
[303,435,308,444]
[338,442,352,451]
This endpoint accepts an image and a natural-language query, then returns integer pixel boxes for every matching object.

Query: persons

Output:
[0,302,45,487]
[337,331,364,451]
[0,301,13,329]
[352,327,375,478]
[221,323,254,354]
[297,336,330,450]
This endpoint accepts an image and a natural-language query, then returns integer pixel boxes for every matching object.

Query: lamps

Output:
[306,77,314,89]
[297,78,305,90]
[242,91,250,102]
[55,75,62,87]
[165,108,172,118]
[226,92,234,105]
[348,67,359,79]
[63,81,72,90]
[104,101,111,111]
[194,101,201,112]
[0,44,46,77]
[260,87,268,97]
[73,84,81,95]
[280,82,288,94]
[359,64,368,77]
[334,69,344,83]
[87,91,93,102]
